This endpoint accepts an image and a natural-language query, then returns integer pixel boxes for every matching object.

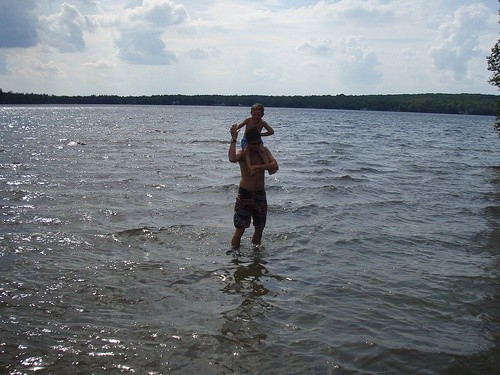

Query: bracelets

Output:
[231,140,236,143]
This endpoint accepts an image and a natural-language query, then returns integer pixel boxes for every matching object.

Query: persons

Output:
[228,127,278,249]
[232,103,278,176]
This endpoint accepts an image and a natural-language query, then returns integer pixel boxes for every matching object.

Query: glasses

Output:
[249,140,263,145]
